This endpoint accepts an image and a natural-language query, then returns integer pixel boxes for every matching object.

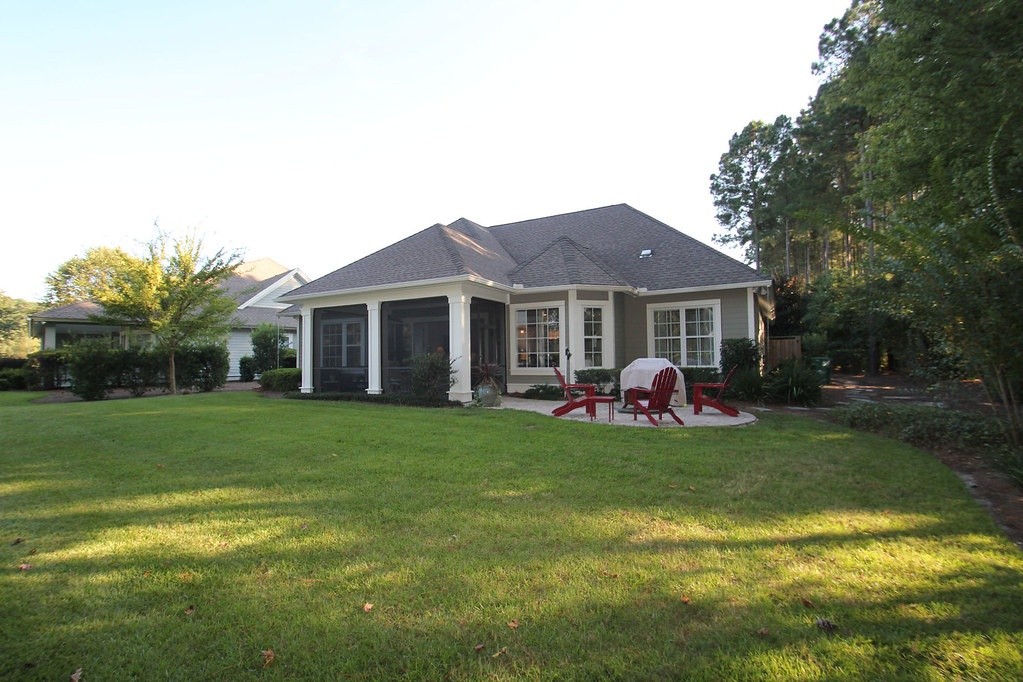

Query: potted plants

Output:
[472,362,505,407]
[326,364,339,381]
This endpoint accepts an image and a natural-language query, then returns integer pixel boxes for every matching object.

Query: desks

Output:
[622,389,650,409]
[587,394,616,423]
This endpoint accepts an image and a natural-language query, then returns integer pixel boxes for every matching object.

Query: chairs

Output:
[626,366,685,427]
[691,363,740,418]
[550,367,596,416]
